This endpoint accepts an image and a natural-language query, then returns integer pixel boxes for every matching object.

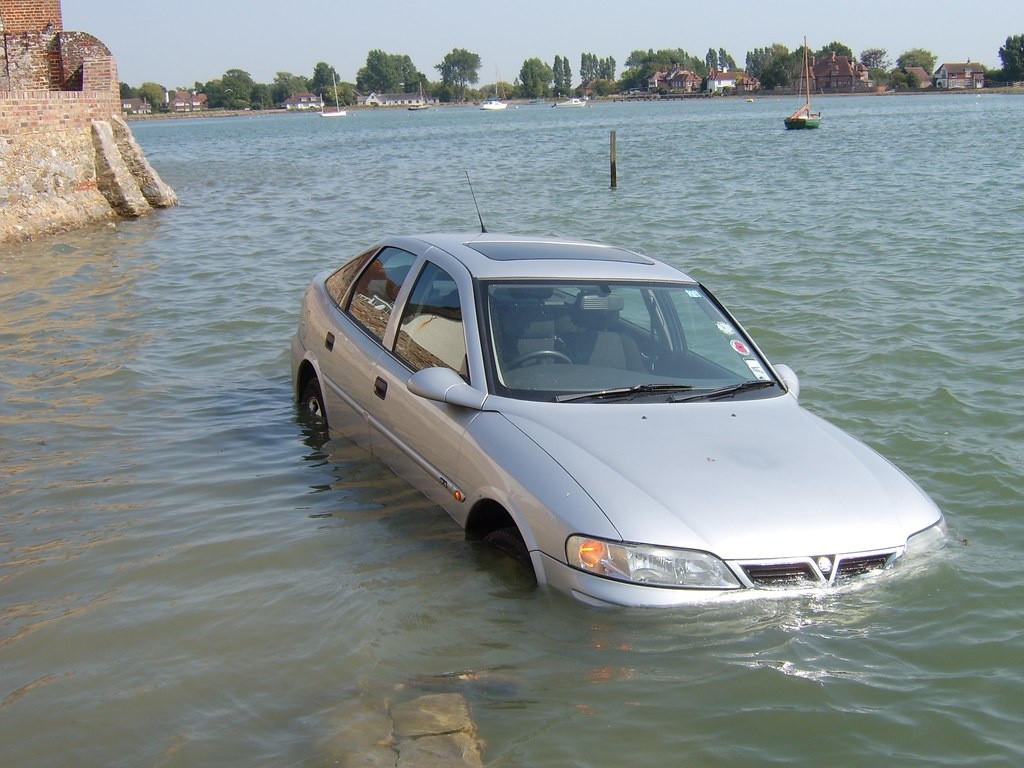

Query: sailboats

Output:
[319,73,348,117]
[405,82,432,110]
[782,35,824,130]
[479,63,509,109]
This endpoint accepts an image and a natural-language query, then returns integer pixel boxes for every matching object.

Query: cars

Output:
[288,231,954,609]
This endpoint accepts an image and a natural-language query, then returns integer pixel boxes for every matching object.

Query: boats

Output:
[550,98,589,107]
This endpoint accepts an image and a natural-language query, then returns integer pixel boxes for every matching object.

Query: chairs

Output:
[571,296,645,373]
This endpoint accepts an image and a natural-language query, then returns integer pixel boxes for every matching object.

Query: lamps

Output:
[24,31,31,38]
[47,19,53,31]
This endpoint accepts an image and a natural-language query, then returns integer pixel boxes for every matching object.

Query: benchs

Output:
[367,279,574,374]
